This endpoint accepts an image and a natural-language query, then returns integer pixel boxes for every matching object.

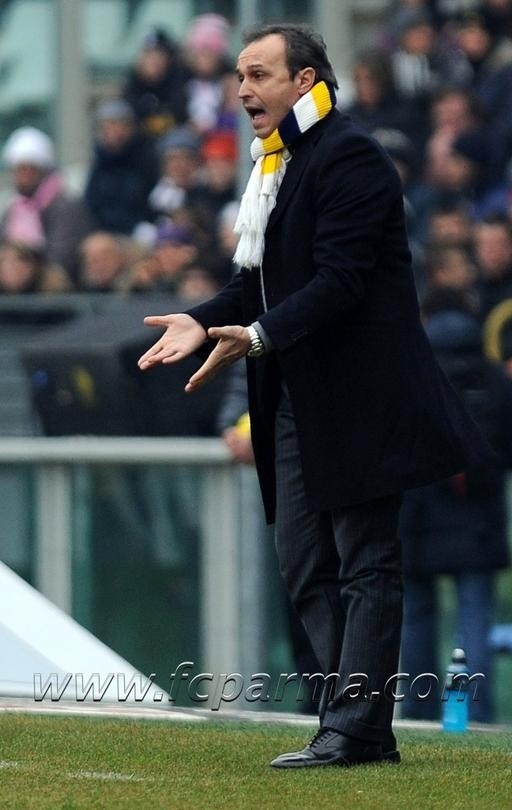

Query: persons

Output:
[131,22,476,772]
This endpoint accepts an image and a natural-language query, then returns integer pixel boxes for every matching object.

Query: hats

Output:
[154,123,241,165]
[2,124,56,169]
[182,11,235,55]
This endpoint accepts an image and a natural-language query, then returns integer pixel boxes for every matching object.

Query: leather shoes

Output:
[270,730,405,769]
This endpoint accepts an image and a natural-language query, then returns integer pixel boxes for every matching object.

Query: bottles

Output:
[438,648,472,732]
